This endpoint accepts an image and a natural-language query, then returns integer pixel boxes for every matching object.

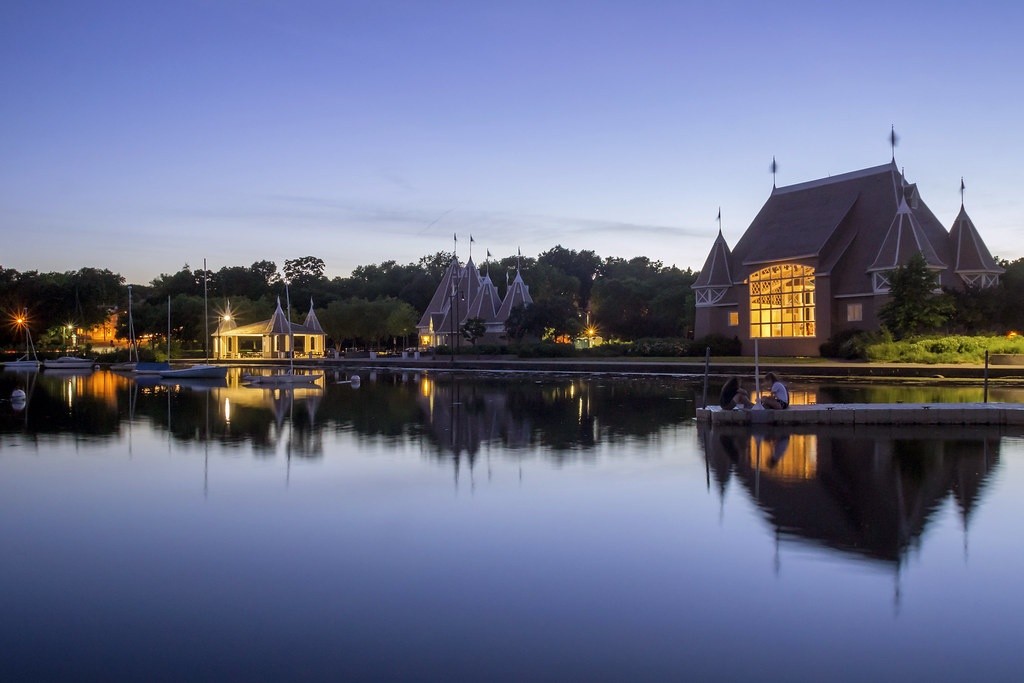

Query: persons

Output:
[719,377,755,410]
[753,372,789,410]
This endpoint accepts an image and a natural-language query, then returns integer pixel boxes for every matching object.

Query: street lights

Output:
[224,313,233,358]
[68,325,74,352]
[15,315,31,360]
[456,290,466,351]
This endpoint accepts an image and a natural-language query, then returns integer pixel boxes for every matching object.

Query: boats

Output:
[42,355,96,368]
[43,368,92,377]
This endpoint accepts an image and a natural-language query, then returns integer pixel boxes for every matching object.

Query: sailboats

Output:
[111,286,139,371]
[116,370,138,452]
[0,306,40,366]
[159,378,228,498]
[4,366,39,426]
[243,381,322,489]
[157,257,228,377]
[240,279,322,381]
[131,295,173,373]
[131,372,171,452]
